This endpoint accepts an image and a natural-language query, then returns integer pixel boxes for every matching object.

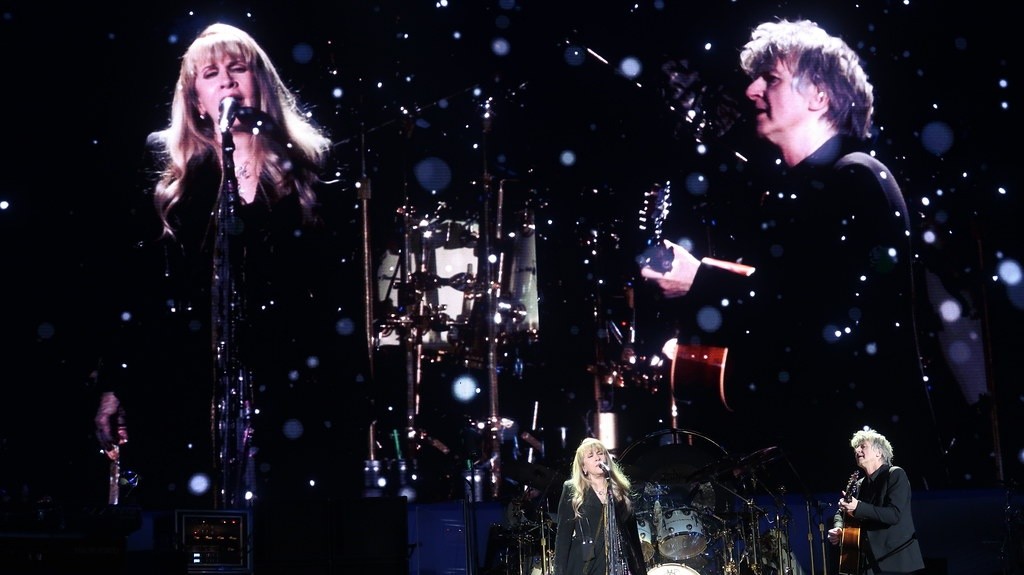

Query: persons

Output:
[98,20,374,535]
[828,431,926,575]
[639,22,927,449]
[553,437,648,575]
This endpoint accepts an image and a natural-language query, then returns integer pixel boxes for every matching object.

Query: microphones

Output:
[599,464,609,476]
[220,96,239,133]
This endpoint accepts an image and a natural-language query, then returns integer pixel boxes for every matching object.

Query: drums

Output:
[364,195,545,348]
[449,467,491,502]
[359,456,416,503]
[656,503,710,561]
[632,515,655,564]
[646,559,701,575]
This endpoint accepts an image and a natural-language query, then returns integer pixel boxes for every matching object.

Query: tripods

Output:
[367,99,557,505]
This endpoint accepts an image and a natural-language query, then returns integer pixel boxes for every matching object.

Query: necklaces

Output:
[594,483,606,496]
[232,146,255,179]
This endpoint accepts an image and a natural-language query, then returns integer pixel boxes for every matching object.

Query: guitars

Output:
[838,468,866,575]
[640,177,761,417]
